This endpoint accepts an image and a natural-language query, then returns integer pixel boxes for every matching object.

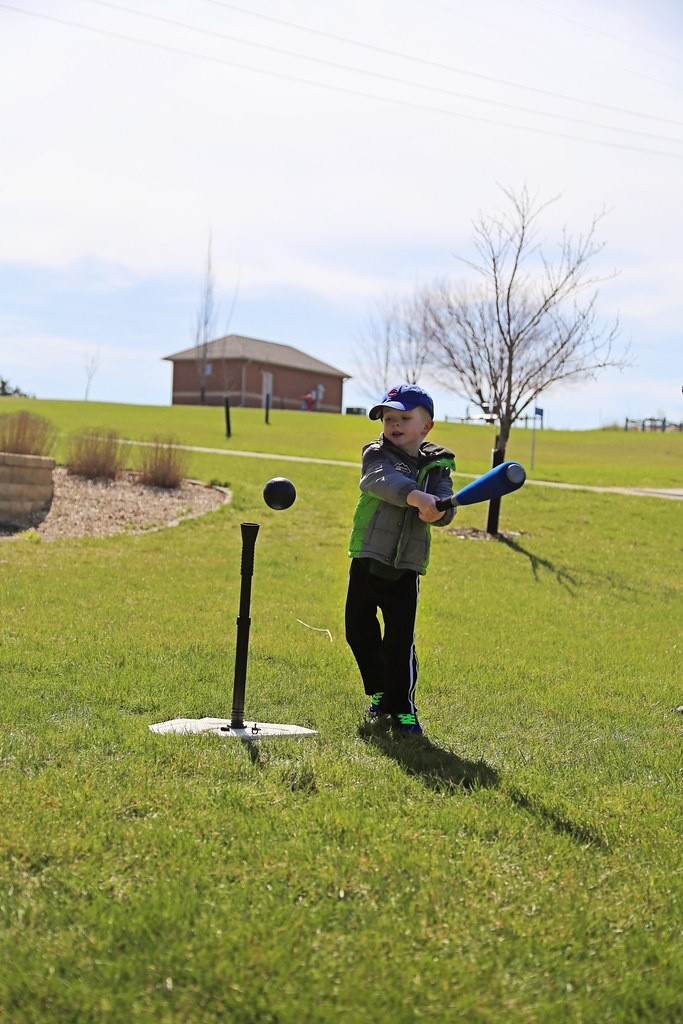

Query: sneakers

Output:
[369,692,384,715]
[392,713,422,735]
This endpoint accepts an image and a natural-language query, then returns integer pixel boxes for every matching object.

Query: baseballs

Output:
[263,477,297,511]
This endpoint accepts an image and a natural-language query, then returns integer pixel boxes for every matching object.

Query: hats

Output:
[369,385,434,421]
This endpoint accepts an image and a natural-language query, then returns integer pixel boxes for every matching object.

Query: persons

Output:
[345,384,457,736]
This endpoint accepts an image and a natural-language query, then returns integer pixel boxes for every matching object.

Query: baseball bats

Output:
[435,460,527,511]
